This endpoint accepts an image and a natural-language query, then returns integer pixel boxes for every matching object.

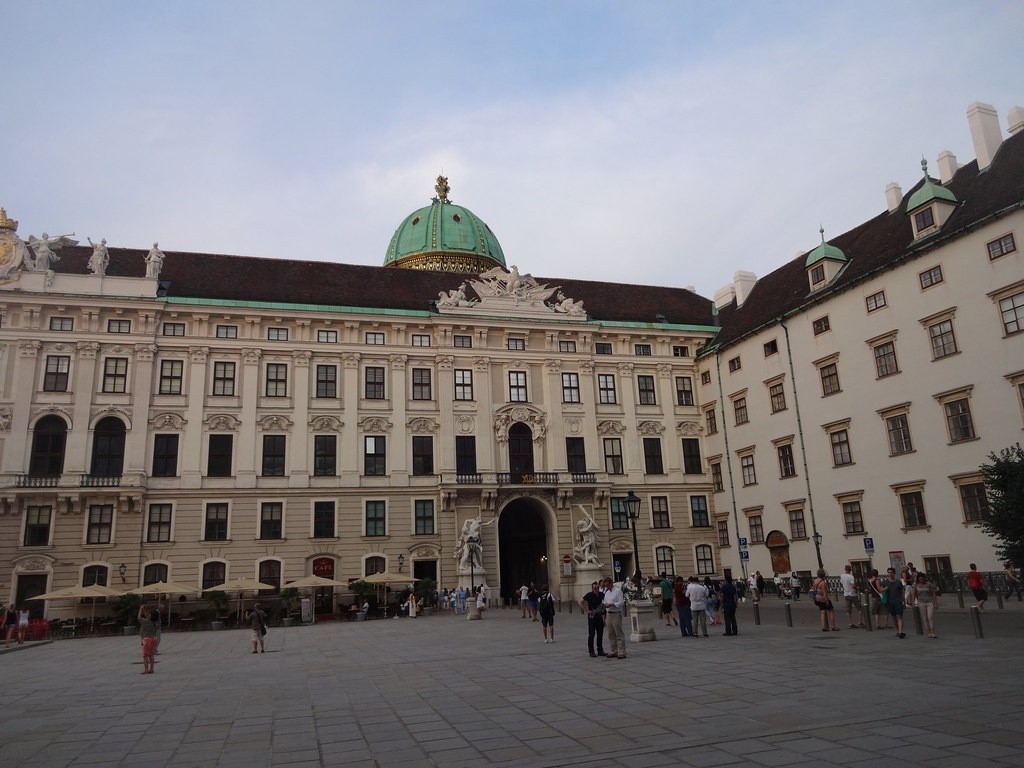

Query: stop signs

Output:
[563,555,571,563]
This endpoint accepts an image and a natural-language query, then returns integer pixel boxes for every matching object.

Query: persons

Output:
[245,603,267,654]
[1,604,30,648]
[362,599,368,613]
[774,571,801,600]
[967,564,987,610]
[748,571,765,602]
[138,604,165,673]
[145,242,165,278]
[643,571,738,637]
[23,233,64,270]
[869,562,938,638]
[573,520,602,568]
[459,518,495,570]
[813,569,840,631]
[1004,564,1022,601]
[519,582,556,643]
[87,237,110,276]
[840,565,864,628]
[402,583,487,618]
[577,578,626,659]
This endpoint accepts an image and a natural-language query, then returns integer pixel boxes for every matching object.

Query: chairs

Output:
[49,603,408,637]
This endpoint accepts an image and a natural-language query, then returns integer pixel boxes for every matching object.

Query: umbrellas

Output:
[26,571,420,636]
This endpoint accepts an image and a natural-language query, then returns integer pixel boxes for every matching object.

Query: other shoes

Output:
[822,622,936,639]
[704,635,708,637]
[666,623,671,627]
[691,634,699,638]
[590,652,596,657]
[533,618,539,622]
[261,650,264,653]
[618,654,626,659]
[598,650,607,656]
[673,618,678,625]
[544,639,548,643]
[607,654,617,657]
[551,639,557,643]
[723,632,732,636]
[252,650,258,653]
[710,620,714,626]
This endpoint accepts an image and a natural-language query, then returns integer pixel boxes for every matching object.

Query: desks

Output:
[178,618,195,631]
[100,623,116,633]
[291,612,300,623]
[378,607,389,618]
[62,625,79,638]
[218,616,229,626]
[348,608,358,618]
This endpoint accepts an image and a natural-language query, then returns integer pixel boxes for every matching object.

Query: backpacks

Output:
[539,594,551,615]
[704,586,717,604]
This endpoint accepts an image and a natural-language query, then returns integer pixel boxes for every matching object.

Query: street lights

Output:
[465,536,478,597]
[812,531,825,570]
[620,489,649,601]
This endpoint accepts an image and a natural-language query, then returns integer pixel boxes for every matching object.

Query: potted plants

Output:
[206,590,230,630]
[279,587,299,626]
[415,577,438,616]
[110,594,141,635]
[347,581,371,622]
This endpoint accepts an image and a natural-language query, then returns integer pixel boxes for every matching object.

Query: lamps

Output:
[398,554,404,571]
[119,563,126,584]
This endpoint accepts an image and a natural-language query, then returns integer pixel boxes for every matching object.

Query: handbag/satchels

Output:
[261,626,266,635]
[879,592,883,599]
